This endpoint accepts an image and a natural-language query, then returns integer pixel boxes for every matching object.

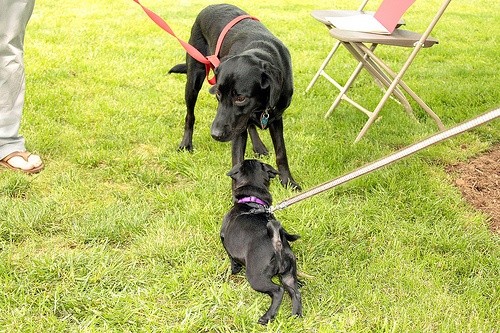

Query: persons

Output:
[0,0,44,173]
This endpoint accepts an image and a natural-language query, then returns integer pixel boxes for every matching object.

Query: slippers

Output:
[0,151,45,173]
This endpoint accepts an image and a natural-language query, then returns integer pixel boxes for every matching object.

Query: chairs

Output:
[305,1,412,121]
[324,1,444,148]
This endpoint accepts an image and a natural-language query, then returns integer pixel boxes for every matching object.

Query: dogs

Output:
[220,159,315,325]
[168,4,303,192]
